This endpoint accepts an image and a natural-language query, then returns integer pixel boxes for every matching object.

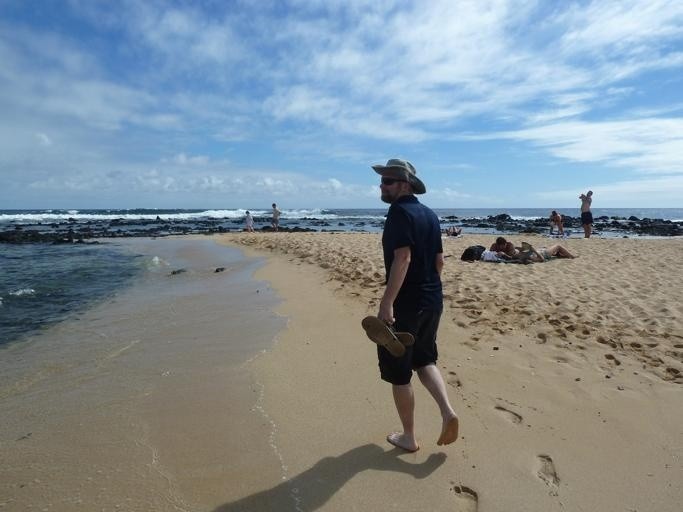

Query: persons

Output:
[444,225,462,236]
[579,191,593,238]
[245,211,255,233]
[489,236,531,256]
[270,203,281,232]
[372,159,459,452]
[547,211,564,236]
[494,244,581,264]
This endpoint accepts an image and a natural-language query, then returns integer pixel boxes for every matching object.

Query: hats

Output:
[371,159,426,194]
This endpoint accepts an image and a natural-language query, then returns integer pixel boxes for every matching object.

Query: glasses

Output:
[381,177,402,184]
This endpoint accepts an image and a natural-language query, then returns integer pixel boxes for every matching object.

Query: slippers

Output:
[361,316,415,357]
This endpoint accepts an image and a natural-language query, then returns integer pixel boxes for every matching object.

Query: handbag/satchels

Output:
[461,245,485,261]
[482,250,498,262]
